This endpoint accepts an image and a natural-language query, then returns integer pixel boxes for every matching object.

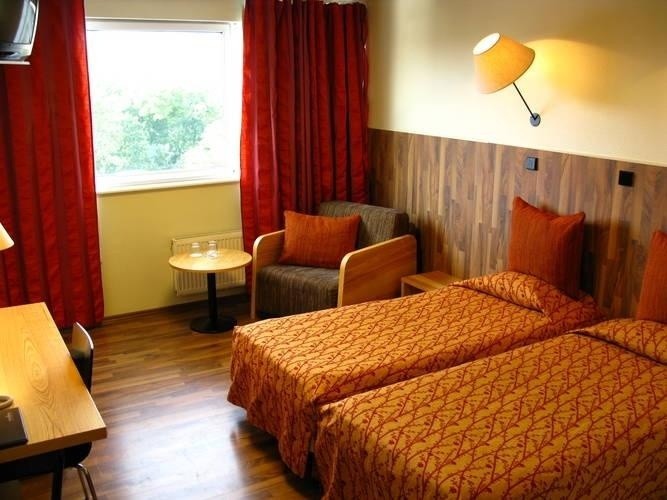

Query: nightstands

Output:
[401,270,464,295]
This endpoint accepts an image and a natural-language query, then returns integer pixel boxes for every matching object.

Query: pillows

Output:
[636,229,666,320]
[566,318,667,363]
[450,271,583,325]
[276,210,361,268]
[508,197,586,300]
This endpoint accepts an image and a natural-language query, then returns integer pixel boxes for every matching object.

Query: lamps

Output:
[472,32,541,126]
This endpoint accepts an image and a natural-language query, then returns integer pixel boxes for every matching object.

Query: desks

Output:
[0,301,108,499]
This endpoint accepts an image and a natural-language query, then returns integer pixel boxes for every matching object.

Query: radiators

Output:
[171,231,245,297]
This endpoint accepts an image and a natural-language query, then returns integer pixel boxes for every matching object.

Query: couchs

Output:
[251,201,417,320]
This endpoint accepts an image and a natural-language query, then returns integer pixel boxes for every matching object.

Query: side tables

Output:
[168,249,252,334]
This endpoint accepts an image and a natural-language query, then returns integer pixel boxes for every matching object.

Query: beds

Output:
[225,268,605,479]
[312,322,667,499]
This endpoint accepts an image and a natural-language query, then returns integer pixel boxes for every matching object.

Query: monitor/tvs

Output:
[0,0,40,62]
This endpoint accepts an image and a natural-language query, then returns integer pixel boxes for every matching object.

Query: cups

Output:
[189,241,202,258]
[207,241,217,258]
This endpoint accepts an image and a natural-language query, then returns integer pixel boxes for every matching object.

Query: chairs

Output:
[0,322,97,499]
